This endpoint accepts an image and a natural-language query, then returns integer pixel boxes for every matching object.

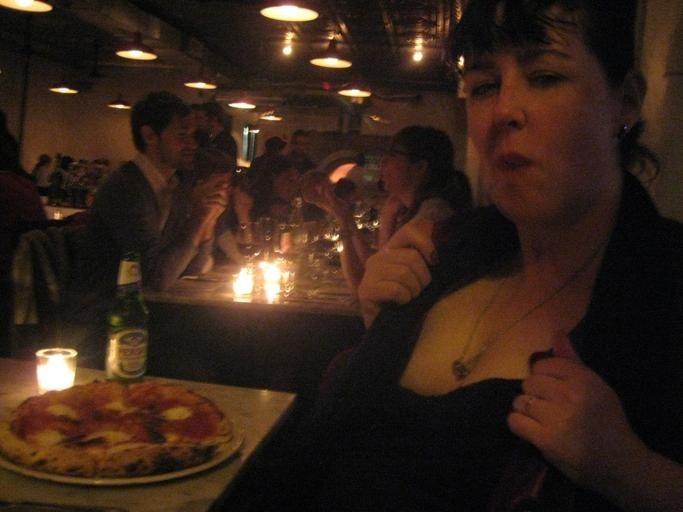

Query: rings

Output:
[522,397,541,416]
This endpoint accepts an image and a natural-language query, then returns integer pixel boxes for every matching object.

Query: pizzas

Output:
[0,383,234,479]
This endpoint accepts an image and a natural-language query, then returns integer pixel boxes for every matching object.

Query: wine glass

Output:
[236,218,335,301]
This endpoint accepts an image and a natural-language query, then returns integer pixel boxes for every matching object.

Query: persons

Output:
[213,2,683,512]
[0,107,48,356]
[69,89,306,384]
[300,124,473,293]
[30,153,113,209]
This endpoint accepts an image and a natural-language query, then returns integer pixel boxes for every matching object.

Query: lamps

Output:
[115,0,158,61]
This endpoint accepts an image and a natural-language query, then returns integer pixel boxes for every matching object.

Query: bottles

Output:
[104,246,153,382]
[284,193,309,238]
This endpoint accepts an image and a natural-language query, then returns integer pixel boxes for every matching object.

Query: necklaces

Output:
[450,231,607,378]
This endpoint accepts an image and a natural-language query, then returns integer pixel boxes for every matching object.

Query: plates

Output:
[0,391,244,486]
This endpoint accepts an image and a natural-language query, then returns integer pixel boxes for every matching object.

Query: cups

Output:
[37,348,77,399]
